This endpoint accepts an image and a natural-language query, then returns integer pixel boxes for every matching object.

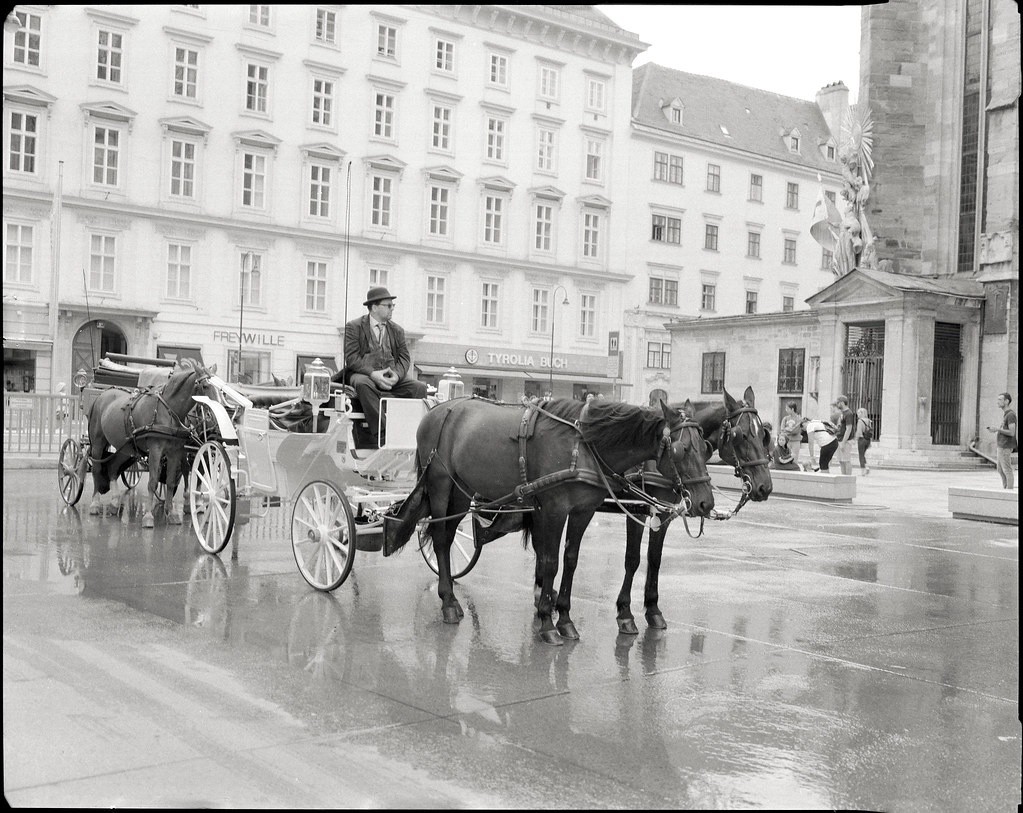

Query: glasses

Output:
[376,303,395,308]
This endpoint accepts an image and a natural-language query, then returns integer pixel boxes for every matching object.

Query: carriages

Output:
[58,352,293,528]
[188,358,772,646]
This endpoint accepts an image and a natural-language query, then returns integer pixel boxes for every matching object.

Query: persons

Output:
[988,392,1017,490]
[345,287,428,440]
[856,408,872,477]
[763,393,854,475]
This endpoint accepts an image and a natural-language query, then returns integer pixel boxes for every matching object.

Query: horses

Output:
[86,362,223,527]
[533,389,772,635]
[414,398,713,645]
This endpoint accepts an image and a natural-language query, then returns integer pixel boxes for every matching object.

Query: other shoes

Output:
[858,467,870,476]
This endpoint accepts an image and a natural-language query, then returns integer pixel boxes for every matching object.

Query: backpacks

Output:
[809,420,841,435]
[860,418,874,440]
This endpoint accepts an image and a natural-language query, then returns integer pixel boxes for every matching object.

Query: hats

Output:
[835,395,848,403]
[363,288,396,305]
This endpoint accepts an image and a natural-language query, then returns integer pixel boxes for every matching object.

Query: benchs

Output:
[705,463,857,504]
[319,381,439,462]
[241,393,305,461]
[947,485,1018,527]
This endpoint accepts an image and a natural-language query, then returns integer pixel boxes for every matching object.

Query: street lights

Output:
[549,286,569,396]
[238,250,260,382]
